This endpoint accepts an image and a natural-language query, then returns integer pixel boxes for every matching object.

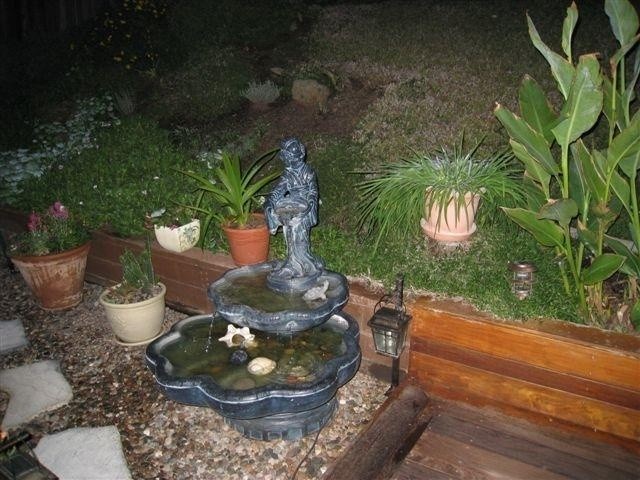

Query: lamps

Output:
[366,294,412,360]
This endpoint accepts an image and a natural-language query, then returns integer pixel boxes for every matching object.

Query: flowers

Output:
[4,197,93,256]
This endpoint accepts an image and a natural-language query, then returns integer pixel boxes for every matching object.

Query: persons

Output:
[263,137,323,279]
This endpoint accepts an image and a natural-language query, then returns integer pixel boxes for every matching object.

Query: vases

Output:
[9,240,91,311]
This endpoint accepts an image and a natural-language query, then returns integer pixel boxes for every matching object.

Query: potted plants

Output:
[347,128,526,262]
[154,143,285,268]
[99,234,168,343]
